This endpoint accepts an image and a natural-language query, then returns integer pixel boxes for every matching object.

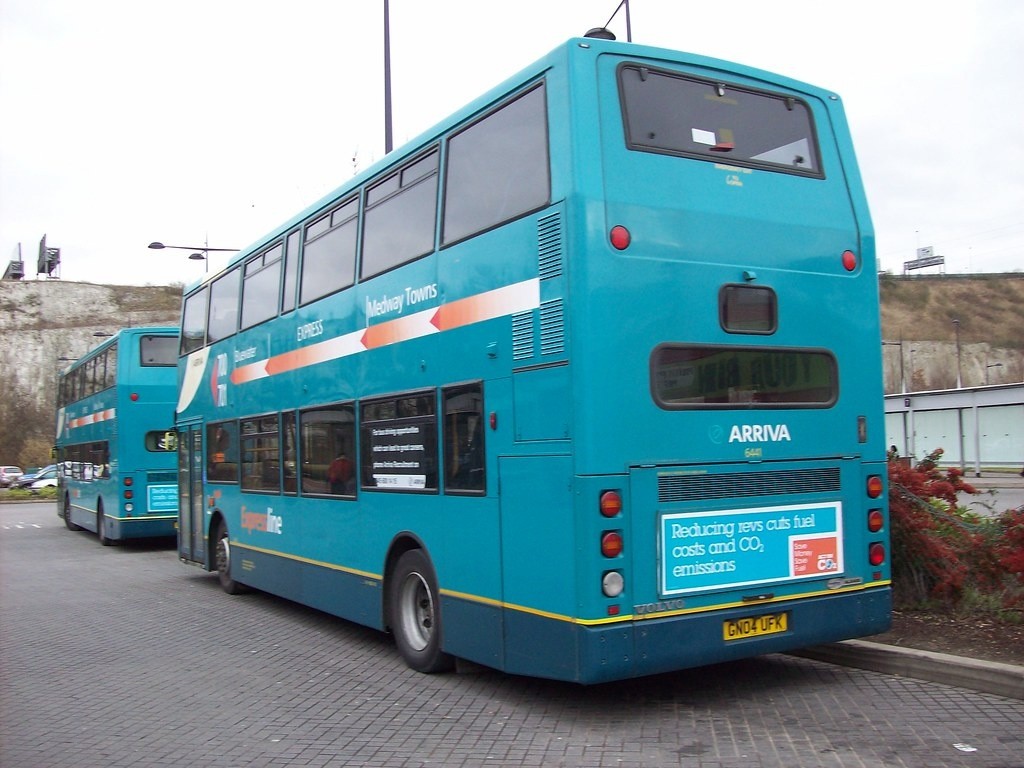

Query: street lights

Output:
[148,242,211,274]
[585,0,633,43]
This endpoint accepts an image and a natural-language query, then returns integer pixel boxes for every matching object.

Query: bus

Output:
[174,0,897,686]
[53,327,187,545]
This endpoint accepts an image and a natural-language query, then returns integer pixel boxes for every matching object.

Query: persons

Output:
[328,453,349,494]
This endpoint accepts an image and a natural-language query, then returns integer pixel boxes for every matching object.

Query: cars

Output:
[9,464,58,492]
[59,463,103,481]
[0,465,24,487]
